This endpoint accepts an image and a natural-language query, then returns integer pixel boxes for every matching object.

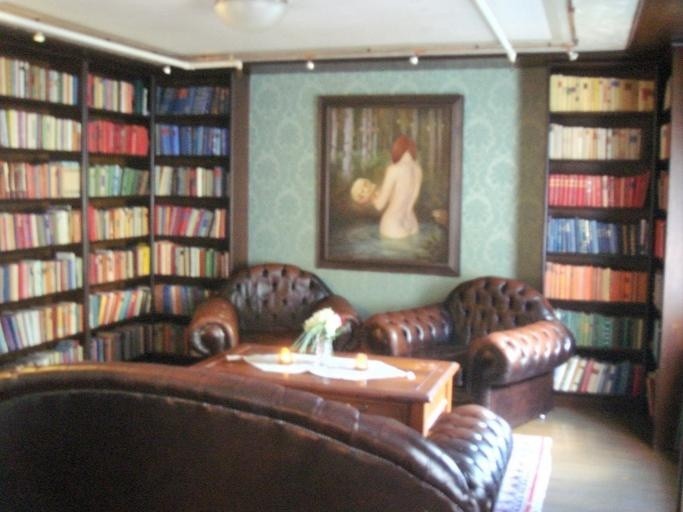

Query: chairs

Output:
[0,365,513,511]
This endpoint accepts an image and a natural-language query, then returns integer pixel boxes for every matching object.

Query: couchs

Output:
[186,263,361,357]
[367,274,573,426]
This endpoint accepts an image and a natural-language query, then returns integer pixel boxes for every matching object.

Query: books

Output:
[86,73,155,361]
[1,58,85,370]
[156,85,230,356]
[545,75,671,259]
[544,263,663,412]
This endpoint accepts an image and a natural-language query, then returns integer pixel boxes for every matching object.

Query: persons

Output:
[370,136,422,238]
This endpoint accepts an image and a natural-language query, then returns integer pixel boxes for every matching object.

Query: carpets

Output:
[501,434,554,511]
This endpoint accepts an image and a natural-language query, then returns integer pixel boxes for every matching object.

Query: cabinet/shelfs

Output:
[0,29,250,378]
[543,43,680,451]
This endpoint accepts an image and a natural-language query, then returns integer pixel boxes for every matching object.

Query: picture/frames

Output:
[318,95,464,278]
